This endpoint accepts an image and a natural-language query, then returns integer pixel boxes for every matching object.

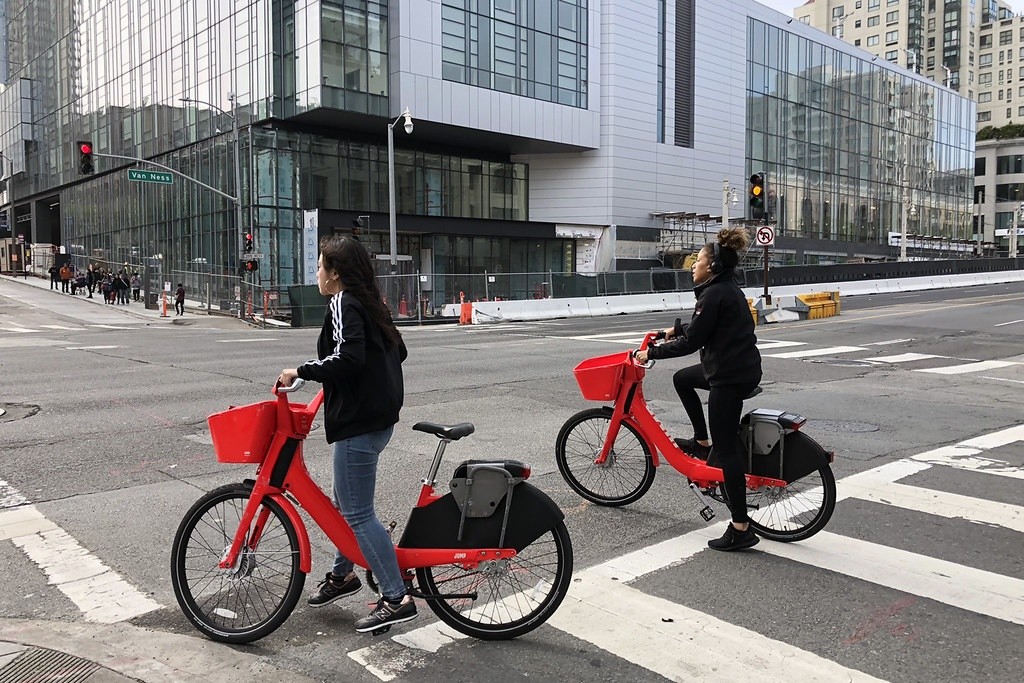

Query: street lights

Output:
[178,96,245,320]
[385,107,415,276]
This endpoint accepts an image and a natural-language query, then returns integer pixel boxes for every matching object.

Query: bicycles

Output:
[556,326,838,541]
[169,367,575,644]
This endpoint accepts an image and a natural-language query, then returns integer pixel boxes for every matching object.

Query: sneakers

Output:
[708,522,759,552]
[354,594,417,632]
[307,571,362,607]
[674,437,710,461]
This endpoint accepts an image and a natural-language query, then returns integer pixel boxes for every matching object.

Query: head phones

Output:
[711,241,725,274]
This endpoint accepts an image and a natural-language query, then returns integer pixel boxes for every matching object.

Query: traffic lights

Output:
[246,261,259,272]
[749,171,768,220]
[76,138,96,175]
[243,232,254,254]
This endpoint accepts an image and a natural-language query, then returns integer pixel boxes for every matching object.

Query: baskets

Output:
[206,399,306,464]
[574,351,628,401]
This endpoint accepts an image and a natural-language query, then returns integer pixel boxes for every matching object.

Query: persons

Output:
[48,262,142,305]
[636,225,761,552]
[174,284,185,316]
[275,234,419,632]
